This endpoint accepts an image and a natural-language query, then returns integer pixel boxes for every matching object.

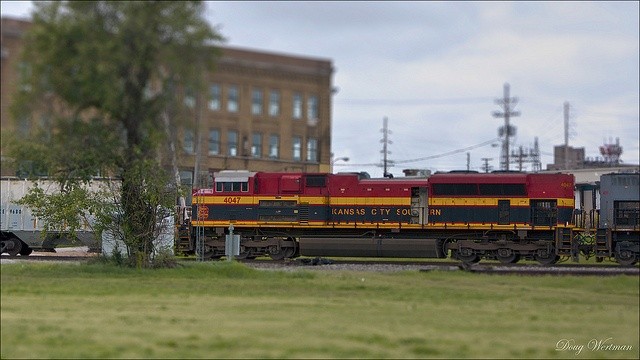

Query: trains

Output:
[0,169,640,266]
